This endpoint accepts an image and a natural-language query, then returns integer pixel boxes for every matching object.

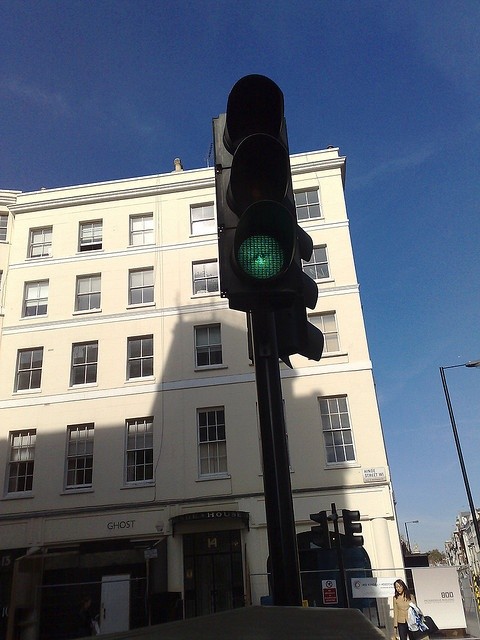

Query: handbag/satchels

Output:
[408,616,439,640]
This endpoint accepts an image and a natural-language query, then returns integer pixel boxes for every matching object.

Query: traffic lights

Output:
[275,222,324,361]
[310,511,330,550]
[342,509,364,546]
[211,73,304,298]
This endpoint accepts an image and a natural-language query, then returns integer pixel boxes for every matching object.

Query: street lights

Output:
[439,359,480,548]
[405,520,419,554]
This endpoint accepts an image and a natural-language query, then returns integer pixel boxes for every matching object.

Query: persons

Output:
[393,579,420,640]
